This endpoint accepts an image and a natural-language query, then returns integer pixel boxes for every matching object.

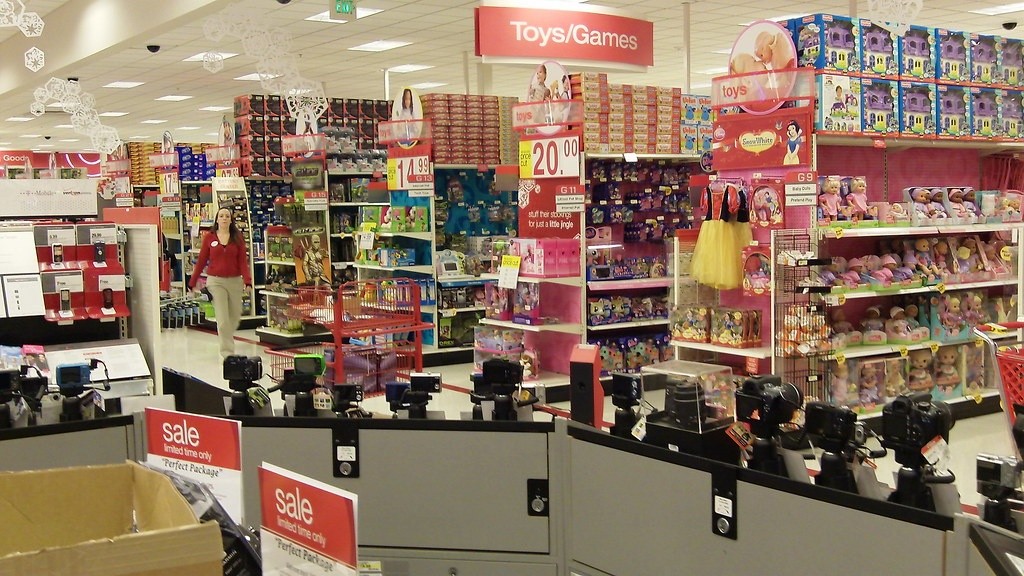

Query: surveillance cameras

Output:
[67,78,78,82]
[277,0,291,4]
[147,45,160,52]
[1003,23,1017,30]
[45,136,51,139]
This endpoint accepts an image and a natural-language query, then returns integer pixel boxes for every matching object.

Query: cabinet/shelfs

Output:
[130,181,266,323]
[674,131,1024,421]
[349,164,519,358]
[255,170,388,347]
[469,154,705,388]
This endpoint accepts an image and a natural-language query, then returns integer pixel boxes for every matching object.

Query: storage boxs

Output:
[123,12,1024,422]
[0,459,227,576]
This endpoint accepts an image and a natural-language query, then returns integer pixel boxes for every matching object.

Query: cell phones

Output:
[60,288,71,311]
[103,288,113,308]
[96,243,105,262]
[52,243,64,263]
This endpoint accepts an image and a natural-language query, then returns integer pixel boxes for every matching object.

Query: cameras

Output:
[56,364,91,396]
[0,369,20,393]
[223,354,262,381]
[285,353,524,403]
[610,372,1024,504]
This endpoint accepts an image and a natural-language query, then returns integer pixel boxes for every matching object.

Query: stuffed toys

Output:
[590,249,673,369]
[474,327,534,378]
[775,314,832,354]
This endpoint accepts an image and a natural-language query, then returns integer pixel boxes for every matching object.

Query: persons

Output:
[720,312,736,338]
[798,27,810,51]
[163,132,172,169]
[398,89,418,146]
[834,86,843,104]
[531,65,568,126]
[189,207,252,360]
[223,122,234,146]
[685,311,698,326]
[730,33,794,111]
[820,178,1023,406]
